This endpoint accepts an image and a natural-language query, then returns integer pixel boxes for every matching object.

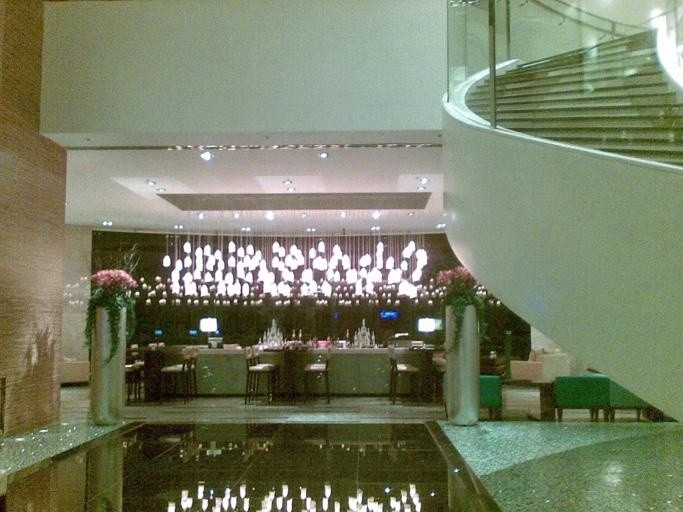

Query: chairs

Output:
[386,345,446,406]
[242,343,332,405]
[124,342,199,405]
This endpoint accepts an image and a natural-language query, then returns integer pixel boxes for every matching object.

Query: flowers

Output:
[436,265,488,352]
[81,268,139,364]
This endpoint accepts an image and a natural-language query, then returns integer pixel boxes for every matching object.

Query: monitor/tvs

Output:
[199,317,218,333]
[416,316,436,333]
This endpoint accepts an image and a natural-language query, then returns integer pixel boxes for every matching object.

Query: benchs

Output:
[477,374,647,423]
